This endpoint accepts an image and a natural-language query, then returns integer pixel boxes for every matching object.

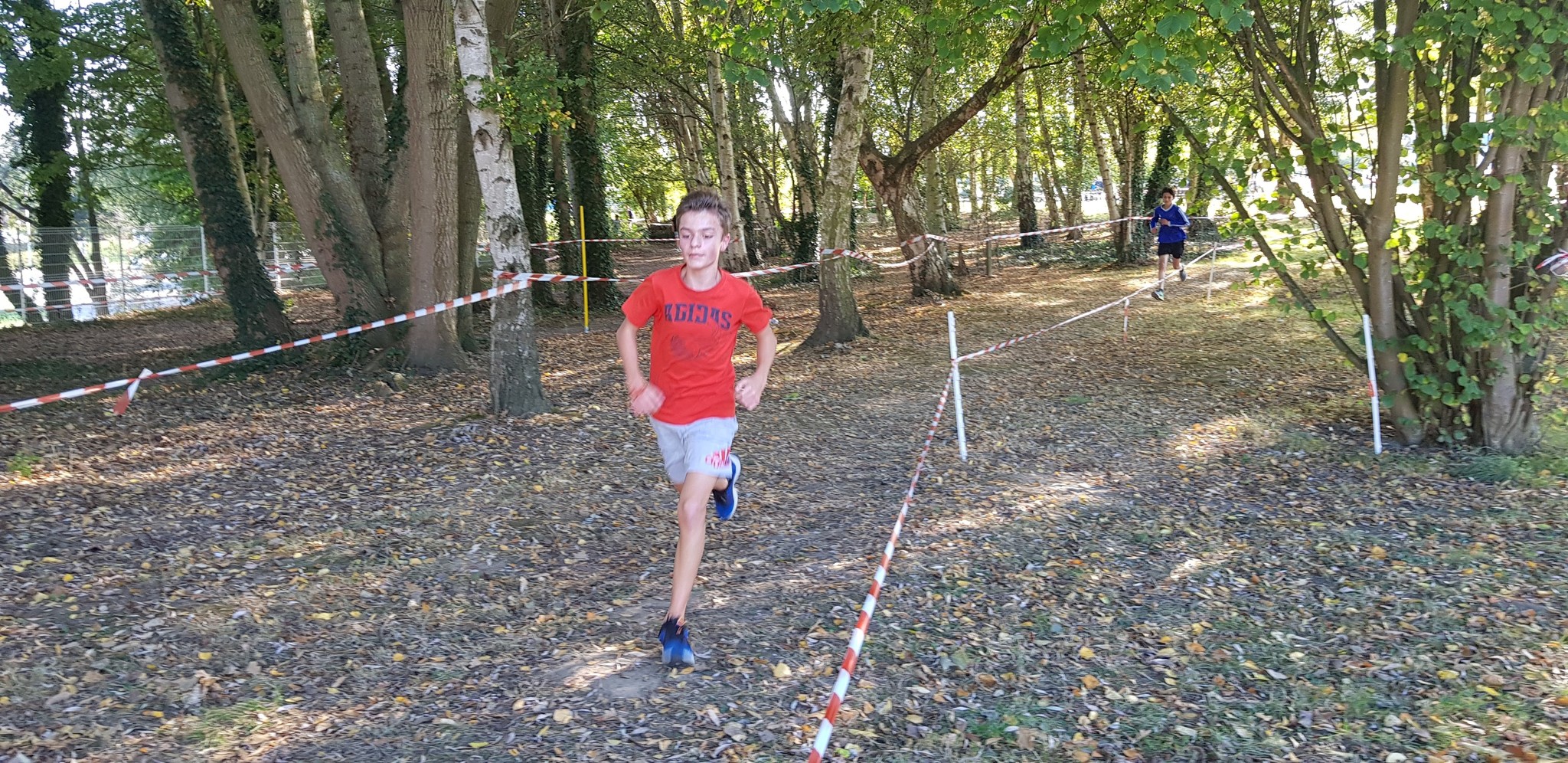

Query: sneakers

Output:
[658,618,696,668]
[1179,262,1186,282]
[1152,290,1164,301]
[712,453,742,521]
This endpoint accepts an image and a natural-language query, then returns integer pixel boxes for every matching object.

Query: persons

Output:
[1149,187,1190,302]
[616,191,777,669]
